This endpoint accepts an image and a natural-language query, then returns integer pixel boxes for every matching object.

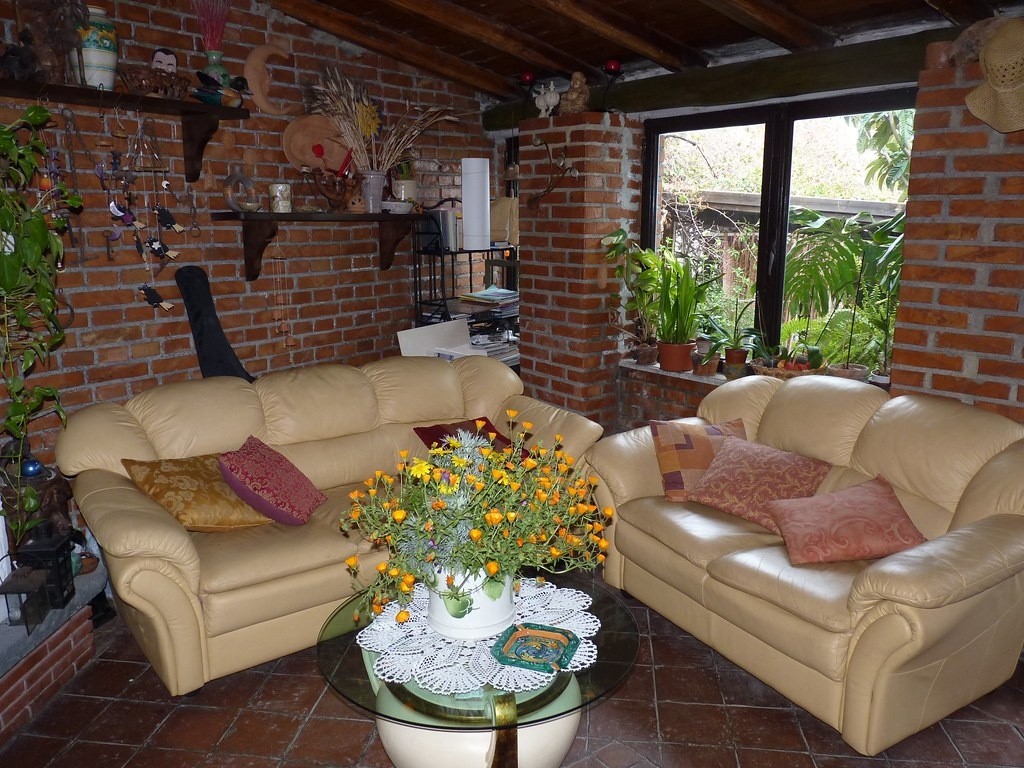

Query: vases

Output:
[357,168,386,217]
[203,51,229,87]
[65,2,117,89]
[427,564,515,641]
[384,214,385,215]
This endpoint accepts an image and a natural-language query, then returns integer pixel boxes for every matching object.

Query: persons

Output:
[559,71,590,114]
[50,0,90,85]
[152,48,177,80]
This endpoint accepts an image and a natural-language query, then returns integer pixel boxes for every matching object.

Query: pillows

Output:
[649,418,747,502]
[317,570,641,768]
[766,474,929,565]
[413,417,539,469]
[121,452,276,533]
[687,432,833,538]
[217,435,328,526]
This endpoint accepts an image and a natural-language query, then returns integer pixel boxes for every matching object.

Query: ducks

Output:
[189,70,254,108]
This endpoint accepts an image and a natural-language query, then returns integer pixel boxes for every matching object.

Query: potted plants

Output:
[602,228,891,392]
[391,158,420,200]
[0,106,85,546]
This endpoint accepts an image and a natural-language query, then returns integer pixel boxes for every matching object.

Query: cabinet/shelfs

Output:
[413,198,517,338]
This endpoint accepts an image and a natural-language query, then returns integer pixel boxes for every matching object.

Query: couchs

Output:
[584,375,1023,756]
[55,355,604,697]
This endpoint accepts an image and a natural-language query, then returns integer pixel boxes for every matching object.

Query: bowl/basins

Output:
[240,202,262,212]
[382,202,415,214]
[121,67,190,100]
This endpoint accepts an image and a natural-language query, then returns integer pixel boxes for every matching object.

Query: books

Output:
[422,285,520,366]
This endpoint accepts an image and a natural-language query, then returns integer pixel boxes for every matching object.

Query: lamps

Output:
[526,137,580,209]
[514,70,540,119]
[594,60,624,112]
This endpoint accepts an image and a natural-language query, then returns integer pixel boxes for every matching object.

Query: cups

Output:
[268,184,292,213]
[392,180,419,204]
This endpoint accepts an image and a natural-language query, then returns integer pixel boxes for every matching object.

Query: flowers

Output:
[338,409,614,623]
